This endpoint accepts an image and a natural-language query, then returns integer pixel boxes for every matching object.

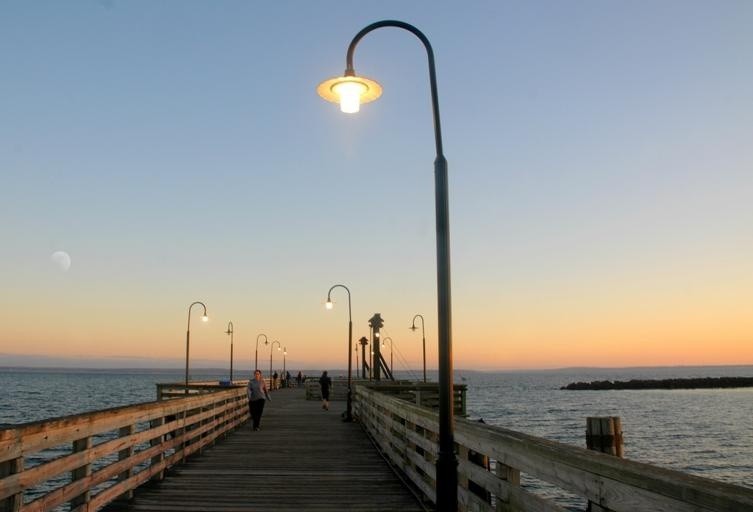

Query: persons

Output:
[247,370,271,432]
[279,370,284,388]
[294,370,301,384]
[302,375,306,387]
[272,370,277,391]
[318,370,331,411]
[285,371,291,388]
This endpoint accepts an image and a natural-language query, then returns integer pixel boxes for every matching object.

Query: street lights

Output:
[353,342,360,377]
[408,314,427,383]
[382,336,393,376]
[315,19,460,512]
[326,284,355,423]
[185,300,287,397]
